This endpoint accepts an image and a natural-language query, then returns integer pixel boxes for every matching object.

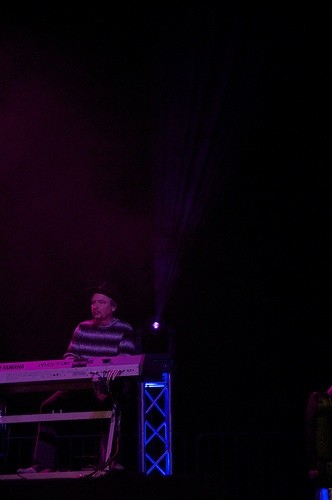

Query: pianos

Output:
[0,354,173,383]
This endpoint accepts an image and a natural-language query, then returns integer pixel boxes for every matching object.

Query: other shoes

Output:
[18,465,54,473]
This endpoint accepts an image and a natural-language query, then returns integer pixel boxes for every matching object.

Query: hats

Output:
[85,281,123,305]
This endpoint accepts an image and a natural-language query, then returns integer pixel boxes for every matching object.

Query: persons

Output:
[16,281,136,475]
